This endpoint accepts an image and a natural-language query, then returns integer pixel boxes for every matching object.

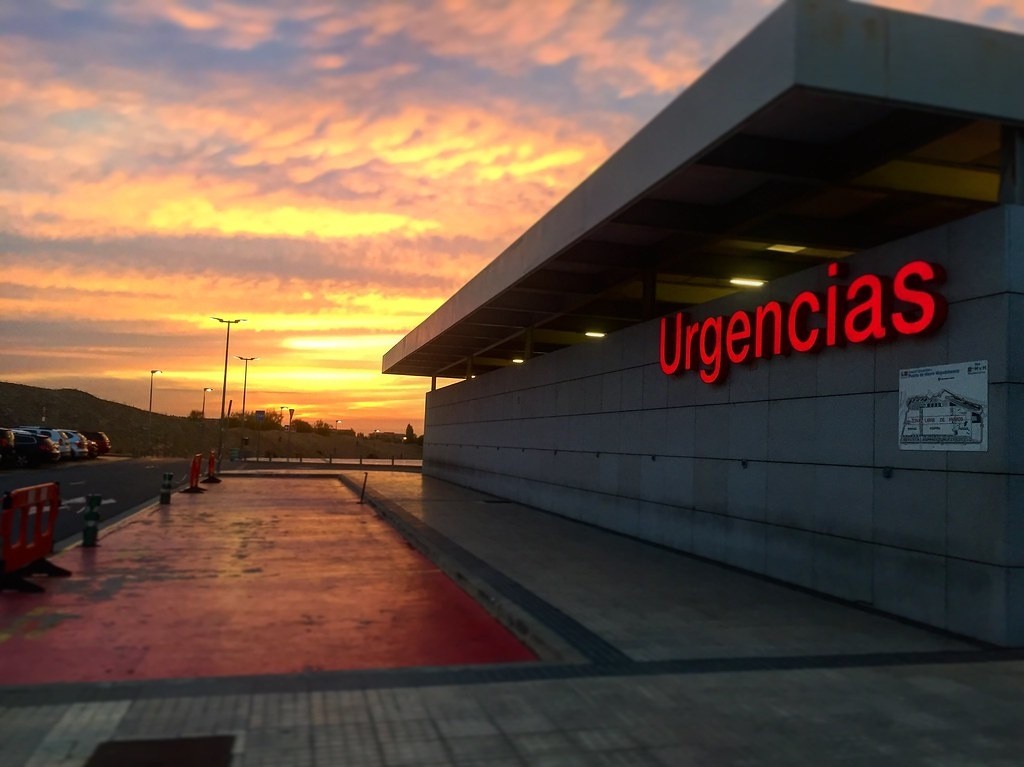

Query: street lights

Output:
[335,420,341,428]
[280,406,288,424]
[210,316,247,474]
[233,355,260,460]
[149,370,163,412]
[202,387,213,415]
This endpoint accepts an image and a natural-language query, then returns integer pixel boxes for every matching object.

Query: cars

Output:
[81,432,112,454]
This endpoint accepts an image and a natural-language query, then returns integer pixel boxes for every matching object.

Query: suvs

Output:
[0,424,97,468]
